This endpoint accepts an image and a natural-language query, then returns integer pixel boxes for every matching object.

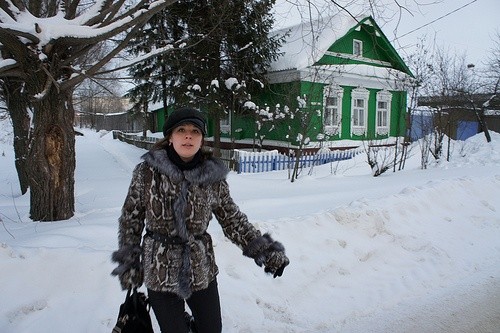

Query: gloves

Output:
[274,263,285,278]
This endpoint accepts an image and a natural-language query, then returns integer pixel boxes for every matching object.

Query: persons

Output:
[110,108,290,333]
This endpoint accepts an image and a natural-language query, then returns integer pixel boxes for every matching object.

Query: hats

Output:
[163,108,207,139]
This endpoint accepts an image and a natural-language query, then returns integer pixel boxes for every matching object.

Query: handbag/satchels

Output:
[112,284,153,332]
